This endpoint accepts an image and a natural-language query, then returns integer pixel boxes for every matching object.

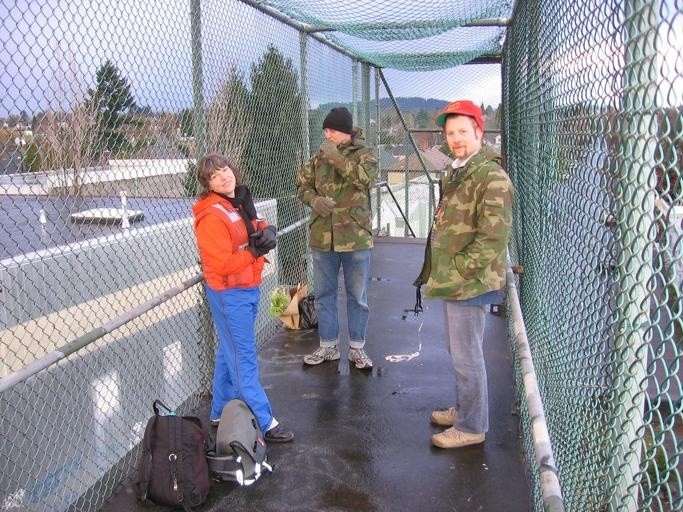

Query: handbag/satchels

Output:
[206,400,272,486]
[299,296,318,329]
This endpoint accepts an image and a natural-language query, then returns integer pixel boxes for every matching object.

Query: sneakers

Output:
[349,349,373,369]
[431,407,485,448]
[304,345,340,365]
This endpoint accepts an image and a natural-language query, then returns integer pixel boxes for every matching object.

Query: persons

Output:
[413,101,514,449]
[192,155,294,444]
[298,106,378,371]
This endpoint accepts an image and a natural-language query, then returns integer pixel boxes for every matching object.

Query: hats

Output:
[322,107,352,134]
[434,101,484,131]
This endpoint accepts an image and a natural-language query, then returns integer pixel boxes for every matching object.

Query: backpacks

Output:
[140,399,210,512]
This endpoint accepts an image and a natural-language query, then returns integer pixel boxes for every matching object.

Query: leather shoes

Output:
[265,426,294,442]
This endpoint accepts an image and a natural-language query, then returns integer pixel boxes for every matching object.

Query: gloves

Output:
[320,140,344,167]
[309,196,336,217]
[245,225,276,257]
